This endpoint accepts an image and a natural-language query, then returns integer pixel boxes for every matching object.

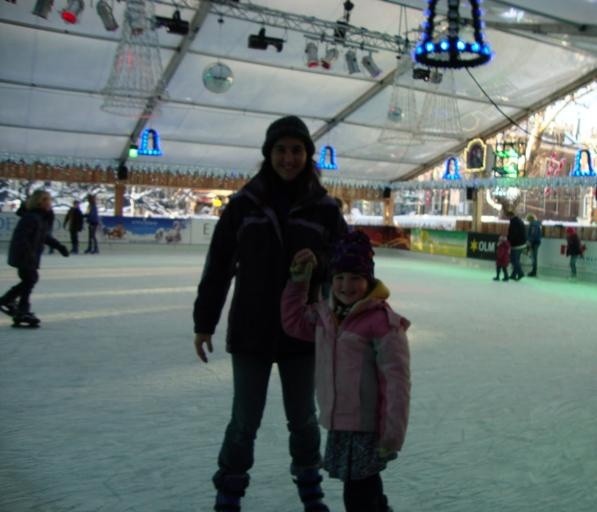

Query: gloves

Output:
[194,333,213,362]
[289,248,320,283]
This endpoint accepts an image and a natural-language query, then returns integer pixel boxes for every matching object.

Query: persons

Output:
[493,235,511,281]
[83,195,99,253]
[0,190,69,325]
[194,115,353,512]
[40,207,54,254]
[506,211,527,280]
[566,226,581,281]
[280,229,411,511]
[63,201,83,253]
[526,213,541,276]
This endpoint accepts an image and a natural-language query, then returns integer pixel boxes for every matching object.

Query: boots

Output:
[2,290,41,325]
[527,267,537,276]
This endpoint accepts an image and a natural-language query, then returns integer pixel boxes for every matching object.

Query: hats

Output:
[498,235,507,240]
[567,227,575,234]
[330,229,374,273]
[263,115,315,156]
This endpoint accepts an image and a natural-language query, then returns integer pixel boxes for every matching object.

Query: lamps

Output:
[128,145,138,158]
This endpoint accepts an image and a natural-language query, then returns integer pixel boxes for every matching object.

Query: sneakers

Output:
[493,272,524,280]
[214,503,241,512]
[305,501,329,512]
[70,247,98,254]
